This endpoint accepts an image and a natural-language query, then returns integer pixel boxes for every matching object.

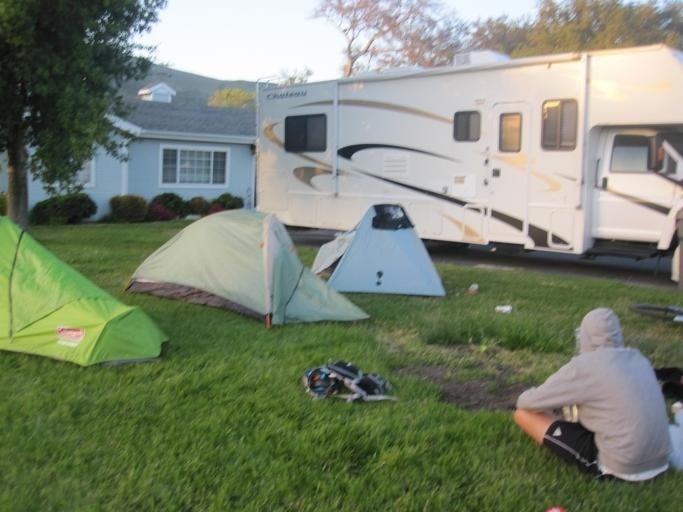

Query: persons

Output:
[514,307,672,482]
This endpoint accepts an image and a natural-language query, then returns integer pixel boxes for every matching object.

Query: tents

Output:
[0,215,171,367]
[310,203,446,296]
[125,209,370,328]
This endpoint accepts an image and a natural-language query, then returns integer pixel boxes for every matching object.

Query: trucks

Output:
[253,43,683,282]
